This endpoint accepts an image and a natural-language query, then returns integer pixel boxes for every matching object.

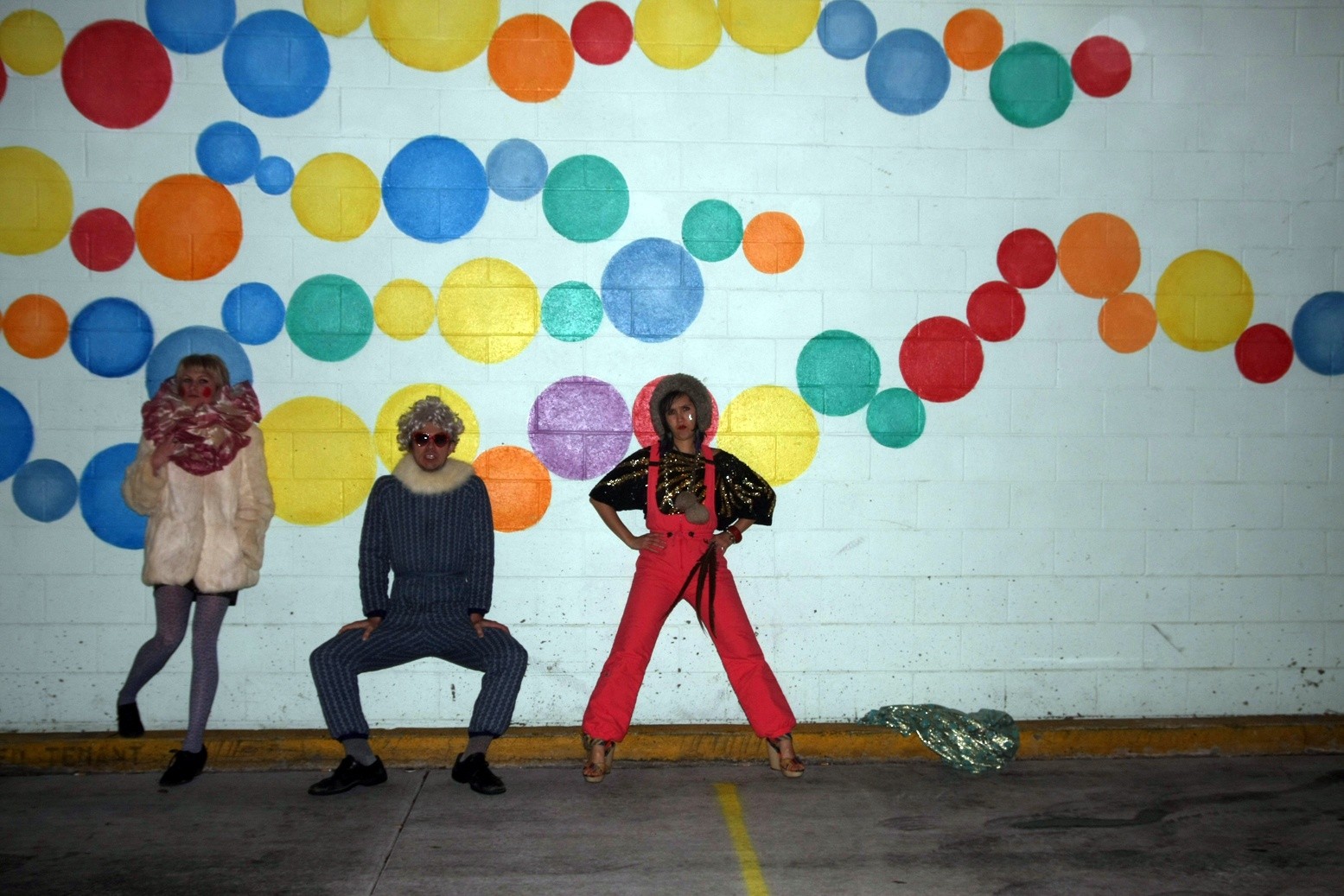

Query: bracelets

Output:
[725,525,743,543]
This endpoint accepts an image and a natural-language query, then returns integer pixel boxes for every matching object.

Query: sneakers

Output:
[453,752,506,795]
[309,755,386,796]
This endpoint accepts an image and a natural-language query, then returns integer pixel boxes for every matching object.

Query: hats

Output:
[651,374,713,440]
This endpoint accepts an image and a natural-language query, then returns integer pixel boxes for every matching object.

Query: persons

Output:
[116,354,277,785]
[581,386,811,783]
[306,399,528,791]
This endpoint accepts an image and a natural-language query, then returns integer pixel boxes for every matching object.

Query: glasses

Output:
[410,431,450,448]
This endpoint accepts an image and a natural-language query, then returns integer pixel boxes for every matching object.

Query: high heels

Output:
[160,742,207,785]
[768,728,805,777]
[118,699,143,737]
[581,732,614,782]
